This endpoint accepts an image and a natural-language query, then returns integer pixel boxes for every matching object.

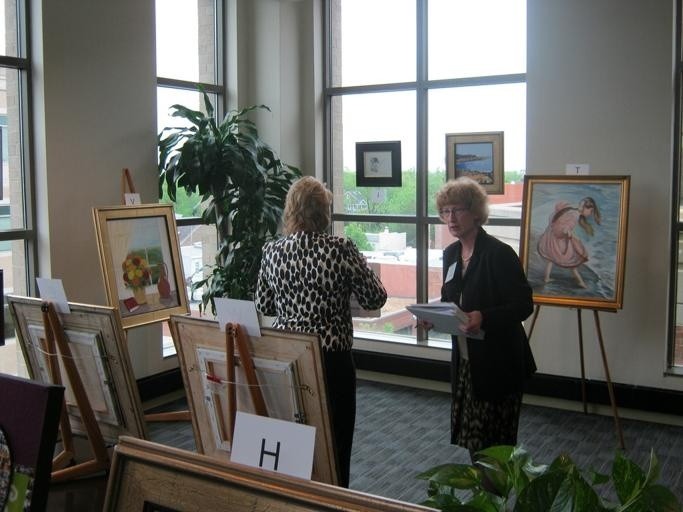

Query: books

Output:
[405,295,489,345]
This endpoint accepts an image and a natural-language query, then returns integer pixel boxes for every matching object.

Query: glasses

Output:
[441,206,469,217]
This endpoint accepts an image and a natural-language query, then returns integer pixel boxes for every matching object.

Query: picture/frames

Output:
[91,202,192,331]
[96,432,444,512]
[354,140,402,188]
[443,130,505,196]
[517,172,630,312]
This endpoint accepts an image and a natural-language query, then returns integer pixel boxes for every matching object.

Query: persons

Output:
[251,175,390,490]
[534,194,601,291]
[414,174,539,495]
